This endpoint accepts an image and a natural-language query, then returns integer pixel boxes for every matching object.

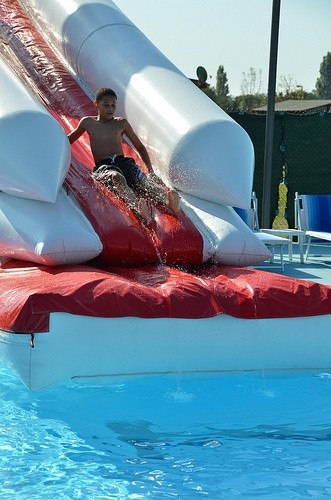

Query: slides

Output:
[0,0,274,267]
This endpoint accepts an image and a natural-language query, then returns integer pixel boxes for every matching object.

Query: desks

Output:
[260,228,305,263]
[252,232,291,272]
[304,231,331,266]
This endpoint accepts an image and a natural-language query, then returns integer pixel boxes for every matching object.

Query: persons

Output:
[68,87,181,226]
